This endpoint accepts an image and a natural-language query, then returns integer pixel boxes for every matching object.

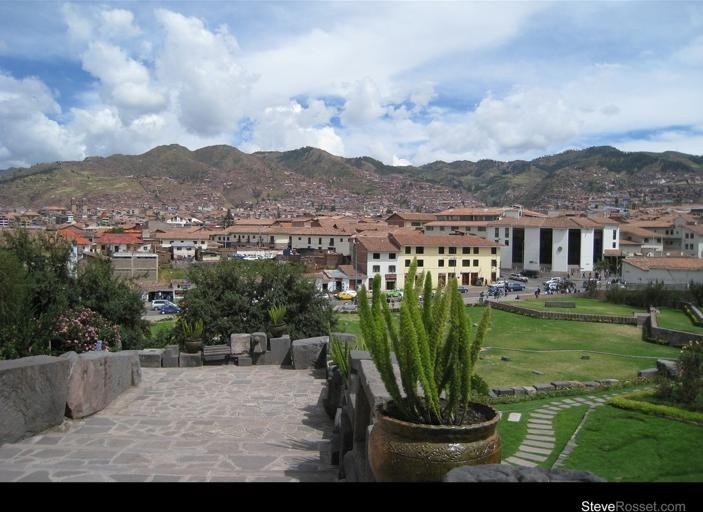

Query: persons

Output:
[477,268,613,302]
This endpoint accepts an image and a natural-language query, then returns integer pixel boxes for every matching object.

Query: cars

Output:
[333,290,357,300]
[387,291,402,302]
[152,300,181,315]
[487,273,528,295]
[457,286,468,293]
[543,277,576,291]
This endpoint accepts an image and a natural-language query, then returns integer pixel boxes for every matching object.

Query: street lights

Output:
[354,238,360,290]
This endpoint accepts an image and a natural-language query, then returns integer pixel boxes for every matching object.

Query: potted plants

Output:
[267,302,288,337]
[356,258,502,482]
[181,318,204,352]
